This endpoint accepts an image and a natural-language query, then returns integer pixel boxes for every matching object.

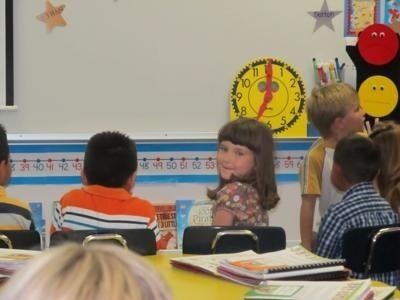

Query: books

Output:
[168,241,398,299]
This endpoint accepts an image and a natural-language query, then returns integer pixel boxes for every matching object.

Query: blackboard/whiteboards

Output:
[0,0,400,144]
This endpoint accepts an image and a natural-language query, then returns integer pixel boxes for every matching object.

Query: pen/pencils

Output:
[313,57,346,87]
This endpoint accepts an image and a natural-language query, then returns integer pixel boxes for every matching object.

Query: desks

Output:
[139,249,400,300]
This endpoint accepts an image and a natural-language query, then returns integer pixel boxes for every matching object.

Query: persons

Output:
[47,128,165,252]
[295,80,380,255]
[310,132,399,292]
[1,239,172,300]
[1,125,42,254]
[204,116,282,229]
[367,124,399,215]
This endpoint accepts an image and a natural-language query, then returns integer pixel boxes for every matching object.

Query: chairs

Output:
[340,226,399,280]
[1,230,41,252]
[48,228,157,256]
[181,227,287,254]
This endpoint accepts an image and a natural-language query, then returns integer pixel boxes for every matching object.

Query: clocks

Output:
[231,55,312,138]
